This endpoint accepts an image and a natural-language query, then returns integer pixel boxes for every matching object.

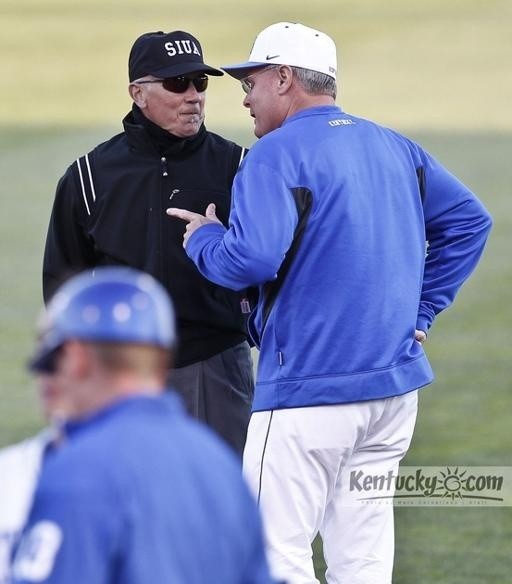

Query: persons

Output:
[0,309,66,583]
[163,19,496,583]
[42,27,259,472]
[0,266,291,583]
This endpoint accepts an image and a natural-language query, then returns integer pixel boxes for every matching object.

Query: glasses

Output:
[238,63,279,94]
[136,74,209,93]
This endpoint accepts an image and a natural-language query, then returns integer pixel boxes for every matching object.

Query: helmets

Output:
[18,265,179,370]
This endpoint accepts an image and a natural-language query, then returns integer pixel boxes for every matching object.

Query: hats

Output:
[128,30,224,83]
[219,21,339,82]
